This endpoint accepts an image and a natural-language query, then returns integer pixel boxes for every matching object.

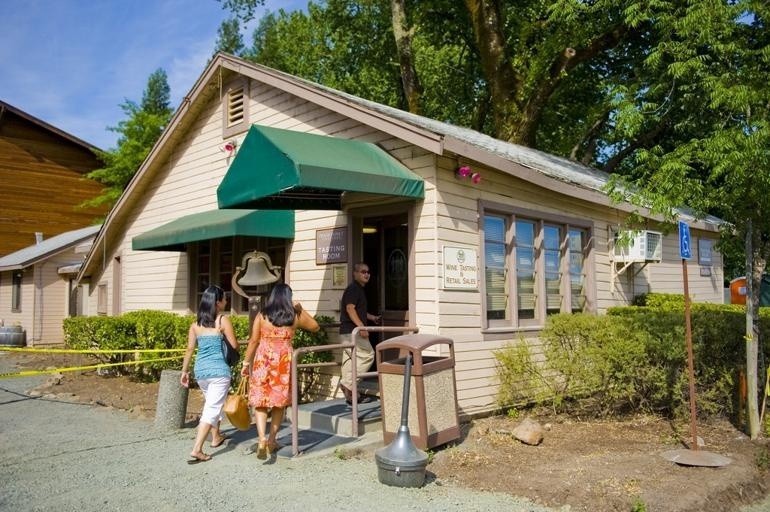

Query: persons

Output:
[241,284,320,460]
[181,285,240,464]
[339,264,380,405]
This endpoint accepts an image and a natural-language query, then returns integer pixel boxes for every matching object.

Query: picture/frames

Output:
[330,263,349,289]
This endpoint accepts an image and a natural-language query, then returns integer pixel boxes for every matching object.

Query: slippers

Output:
[257,439,280,459]
[211,433,229,448]
[187,452,211,464]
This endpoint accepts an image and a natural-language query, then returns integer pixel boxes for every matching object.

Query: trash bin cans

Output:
[730,276,748,305]
[375,333,461,452]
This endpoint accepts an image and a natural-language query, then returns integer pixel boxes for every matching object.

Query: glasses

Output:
[361,269,370,273]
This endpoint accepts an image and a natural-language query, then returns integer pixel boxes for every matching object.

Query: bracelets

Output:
[243,360,250,366]
[182,367,189,373]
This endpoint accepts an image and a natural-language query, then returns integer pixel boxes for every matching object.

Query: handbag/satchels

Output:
[223,394,252,431]
[222,337,240,367]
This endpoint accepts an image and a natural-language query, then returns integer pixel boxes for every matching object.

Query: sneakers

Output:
[339,382,371,405]
